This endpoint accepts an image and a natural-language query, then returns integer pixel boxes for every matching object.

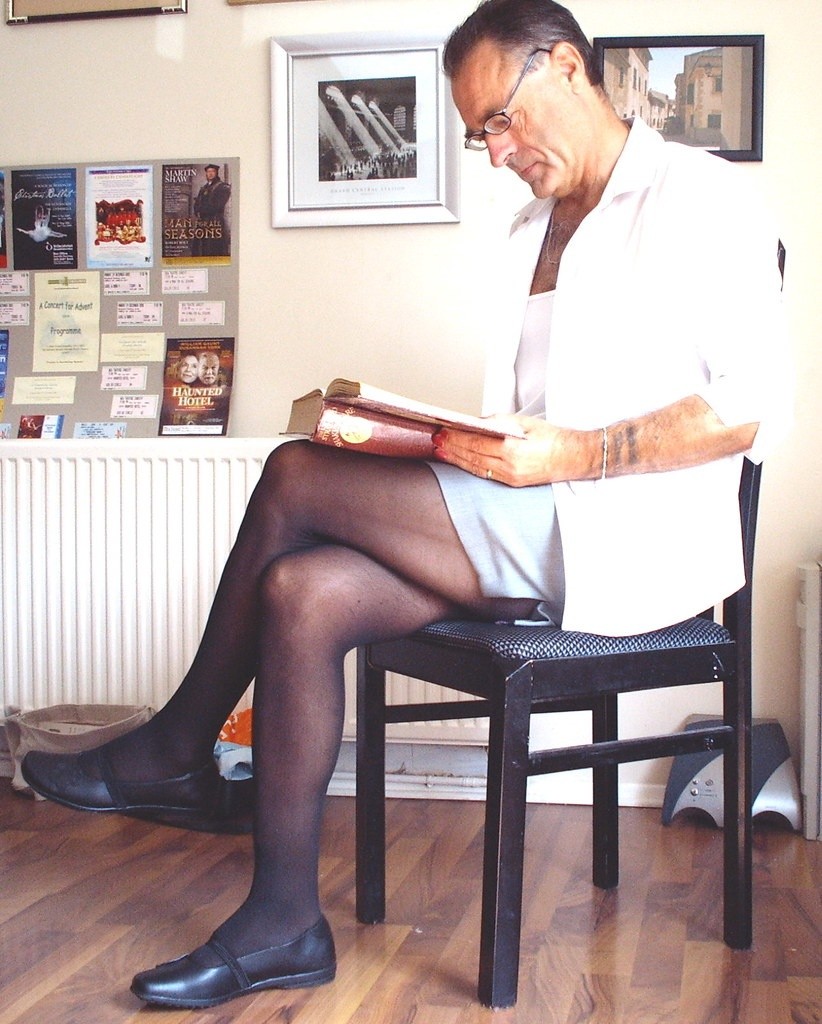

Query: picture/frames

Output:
[269,35,464,228]
[5,0,188,26]
[592,32,766,161]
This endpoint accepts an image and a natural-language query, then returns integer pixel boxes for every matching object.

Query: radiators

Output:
[0,436,492,749]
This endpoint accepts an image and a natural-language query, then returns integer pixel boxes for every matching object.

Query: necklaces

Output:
[546,211,559,264]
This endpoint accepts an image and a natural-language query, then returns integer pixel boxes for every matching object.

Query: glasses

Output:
[465,48,552,151]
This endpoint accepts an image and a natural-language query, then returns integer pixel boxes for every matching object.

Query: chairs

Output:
[359,237,787,1014]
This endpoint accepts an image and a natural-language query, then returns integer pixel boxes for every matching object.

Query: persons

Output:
[191,164,231,257]
[20,0,792,1010]
[176,348,220,387]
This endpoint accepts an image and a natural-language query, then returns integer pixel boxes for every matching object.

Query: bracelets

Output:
[600,427,608,481]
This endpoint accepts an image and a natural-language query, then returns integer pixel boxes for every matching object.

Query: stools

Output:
[661,715,804,830]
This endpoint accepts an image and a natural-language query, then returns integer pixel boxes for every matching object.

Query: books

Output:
[278,378,526,460]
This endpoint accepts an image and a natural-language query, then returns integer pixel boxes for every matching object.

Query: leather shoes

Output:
[130,912,336,1009]
[19,744,224,812]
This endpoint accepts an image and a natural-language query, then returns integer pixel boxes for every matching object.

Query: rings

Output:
[487,470,492,480]
